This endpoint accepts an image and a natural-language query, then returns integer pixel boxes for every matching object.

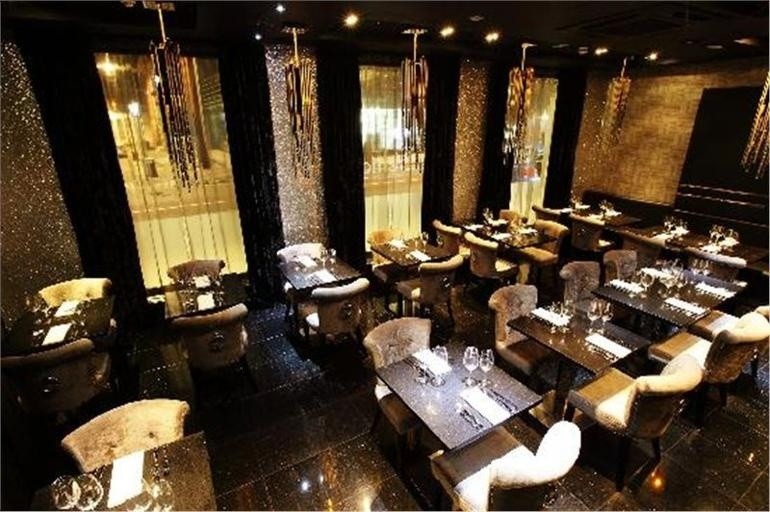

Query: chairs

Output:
[276,242,328,324]
[1,338,111,421]
[173,303,258,396]
[311,276,369,359]
[61,398,191,474]
[38,276,122,344]
[164,260,224,286]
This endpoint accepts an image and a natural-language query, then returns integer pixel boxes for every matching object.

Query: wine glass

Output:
[640,215,734,300]
[52,473,176,512]
[393,231,445,254]
[37,295,52,324]
[26,295,42,325]
[549,297,615,334]
[182,272,226,313]
[318,249,329,270]
[413,345,495,388]
